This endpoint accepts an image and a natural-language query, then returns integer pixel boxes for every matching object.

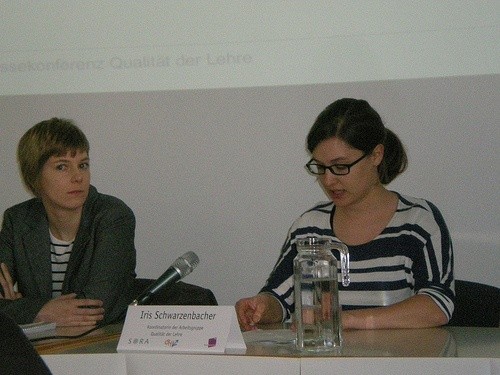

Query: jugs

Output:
[293,237,351,352]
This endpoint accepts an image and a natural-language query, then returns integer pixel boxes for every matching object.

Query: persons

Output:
[0,117,137,326]
[235,98,456,332]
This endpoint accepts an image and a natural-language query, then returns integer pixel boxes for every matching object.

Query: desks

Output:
[25,316,500,375]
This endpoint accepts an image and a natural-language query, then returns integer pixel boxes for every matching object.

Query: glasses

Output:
[306,152,370,176]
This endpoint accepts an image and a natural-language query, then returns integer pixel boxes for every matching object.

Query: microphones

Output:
[128,251,200,308]
